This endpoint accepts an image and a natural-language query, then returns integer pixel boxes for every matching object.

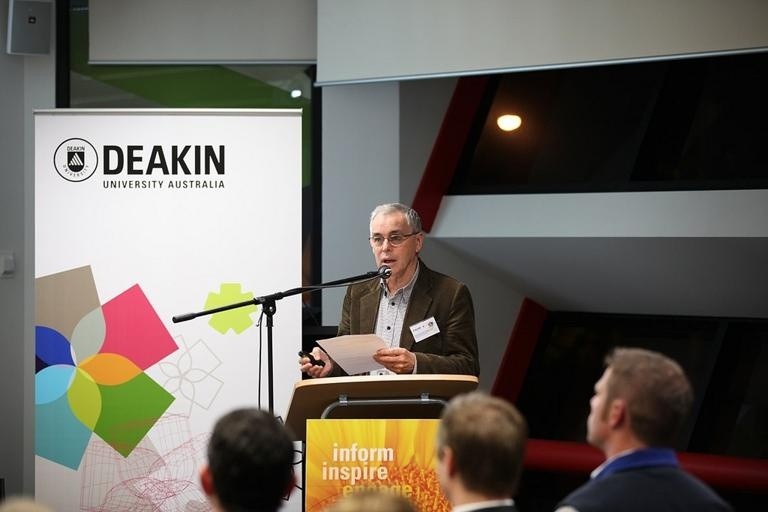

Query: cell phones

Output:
[298,350,325,369]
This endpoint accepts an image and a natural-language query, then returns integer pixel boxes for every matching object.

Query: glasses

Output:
[369,233,419,248]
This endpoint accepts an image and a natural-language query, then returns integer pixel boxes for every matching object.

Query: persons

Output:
[296,201,482,378]
[547,345,732,511]
[198,407,295,511]
[432,390,532,512]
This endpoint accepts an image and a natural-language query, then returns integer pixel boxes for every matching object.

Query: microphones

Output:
[377,264,392,279]
